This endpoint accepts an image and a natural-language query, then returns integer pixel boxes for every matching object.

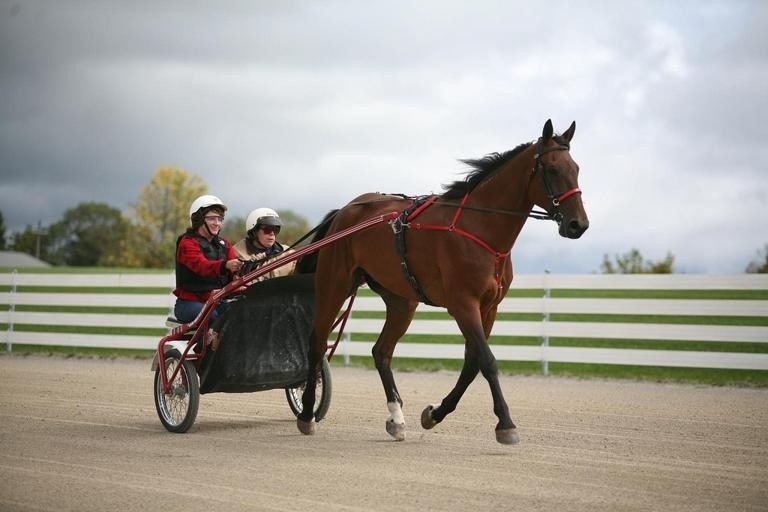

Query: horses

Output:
[290,118,589,444]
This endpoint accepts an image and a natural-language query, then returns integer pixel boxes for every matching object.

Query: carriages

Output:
[148,117,592,448]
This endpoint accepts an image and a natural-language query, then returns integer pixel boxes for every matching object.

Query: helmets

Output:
[189,196,227,224]
[246,209,282,230]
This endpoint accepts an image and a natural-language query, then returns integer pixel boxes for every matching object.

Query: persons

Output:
[172,195,267,343]
[231,207,300,295]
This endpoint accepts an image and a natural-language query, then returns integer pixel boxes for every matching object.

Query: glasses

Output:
[258,227,280,235]
